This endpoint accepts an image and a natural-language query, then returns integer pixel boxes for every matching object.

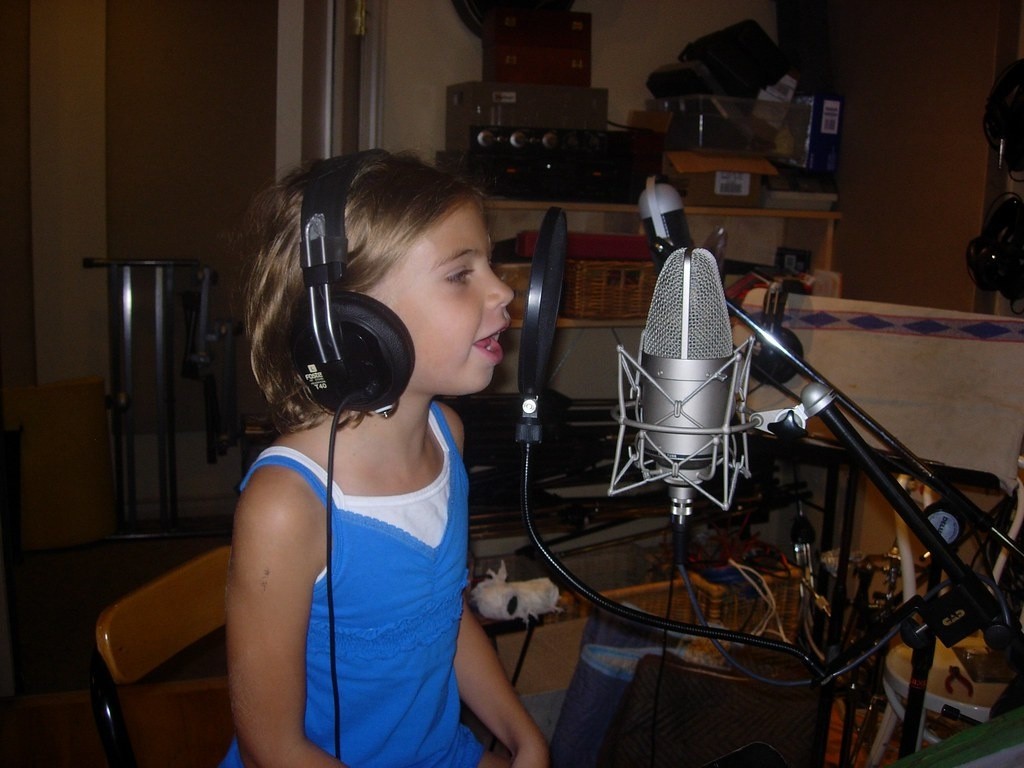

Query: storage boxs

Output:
[628,91,847,205]
[435,11,613,191]
[493,233,664,319]
[514,228,654,260]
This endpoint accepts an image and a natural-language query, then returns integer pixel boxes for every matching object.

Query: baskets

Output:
[493,258,657,320]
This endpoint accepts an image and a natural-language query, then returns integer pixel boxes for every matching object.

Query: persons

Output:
[223,151,548,768]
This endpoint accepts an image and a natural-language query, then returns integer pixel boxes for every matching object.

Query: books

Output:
[761,167,840,210]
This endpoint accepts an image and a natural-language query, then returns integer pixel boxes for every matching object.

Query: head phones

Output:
[292,147,416,416]
[966,191,1024,301]
[983,58,1024,172]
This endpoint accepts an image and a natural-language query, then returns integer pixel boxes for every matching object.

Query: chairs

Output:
[91,543,232,766]
[862,472,1024,766]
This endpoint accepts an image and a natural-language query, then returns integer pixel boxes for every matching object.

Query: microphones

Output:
[638,247,733,535]
[637,184,694,268]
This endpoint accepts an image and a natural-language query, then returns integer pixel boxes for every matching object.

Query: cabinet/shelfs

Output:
[482,202,842,334]
